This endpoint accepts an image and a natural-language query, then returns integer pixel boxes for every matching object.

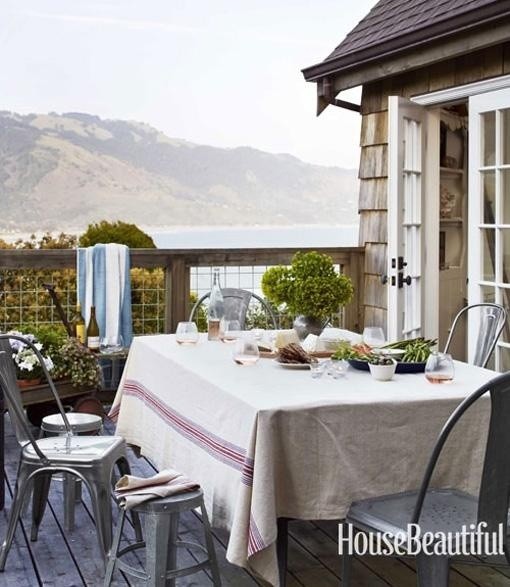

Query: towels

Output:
[114,470,200,510]
[76,243,133,347]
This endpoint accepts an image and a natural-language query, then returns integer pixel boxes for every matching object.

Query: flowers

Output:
[6,330,53,379]
[48,338,104,391]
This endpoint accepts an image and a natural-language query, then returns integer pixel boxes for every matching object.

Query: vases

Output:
[18,380,40,389]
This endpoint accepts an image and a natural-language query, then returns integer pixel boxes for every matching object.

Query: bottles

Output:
[205,267,229,343]
[74,302,101,352]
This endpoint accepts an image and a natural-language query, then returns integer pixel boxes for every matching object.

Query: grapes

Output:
[370,356,394,365]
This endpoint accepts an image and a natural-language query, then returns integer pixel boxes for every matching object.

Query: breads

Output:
[277,342,313,364]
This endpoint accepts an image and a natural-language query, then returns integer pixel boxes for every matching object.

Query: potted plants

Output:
[263,251,354,342]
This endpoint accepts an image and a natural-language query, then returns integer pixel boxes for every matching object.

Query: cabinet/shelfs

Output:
[440,107,468,271]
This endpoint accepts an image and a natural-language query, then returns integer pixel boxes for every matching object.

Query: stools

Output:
[38,413,105,531]
[104,491,224,587]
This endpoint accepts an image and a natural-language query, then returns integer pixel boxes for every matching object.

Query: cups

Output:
[175,318,457,385]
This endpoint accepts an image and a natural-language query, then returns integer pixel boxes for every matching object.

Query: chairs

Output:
[189,288,277,331]
[443,303,507,368]
[0,335,143,580]
[343,372,510,587]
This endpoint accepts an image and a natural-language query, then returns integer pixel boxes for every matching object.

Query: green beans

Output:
[400,339,430,364]
